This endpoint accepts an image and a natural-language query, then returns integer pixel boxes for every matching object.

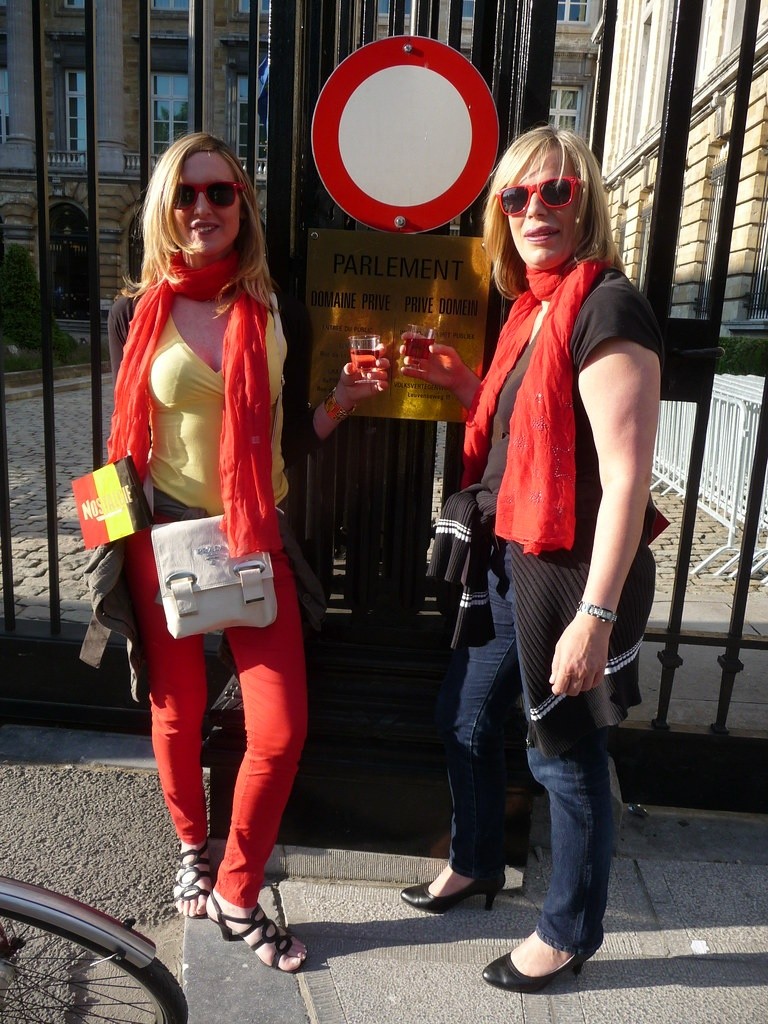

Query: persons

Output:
[396,125,662,994]
[105,132,390,974]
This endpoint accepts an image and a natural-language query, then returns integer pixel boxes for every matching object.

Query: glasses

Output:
[495,176,584,215]
[172,180,246,209]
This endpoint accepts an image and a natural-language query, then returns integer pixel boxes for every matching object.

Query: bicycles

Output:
[0,875,188,1024]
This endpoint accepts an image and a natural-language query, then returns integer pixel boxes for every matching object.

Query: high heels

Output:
[172,835,212,918]
[481,949,595,993]
[401,869,506,914]
[206,890,307,972]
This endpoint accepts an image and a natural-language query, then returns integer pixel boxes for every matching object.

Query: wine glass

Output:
[405,324,437,373]
[348,335,380,384]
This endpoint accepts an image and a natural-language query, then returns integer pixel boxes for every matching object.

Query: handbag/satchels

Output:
[150,513,278,640]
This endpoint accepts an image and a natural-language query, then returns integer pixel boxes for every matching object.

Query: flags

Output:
[71,454,153,550]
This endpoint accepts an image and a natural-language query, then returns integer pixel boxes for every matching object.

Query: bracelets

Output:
[324,386,358,424]
[577,601,617,624]
